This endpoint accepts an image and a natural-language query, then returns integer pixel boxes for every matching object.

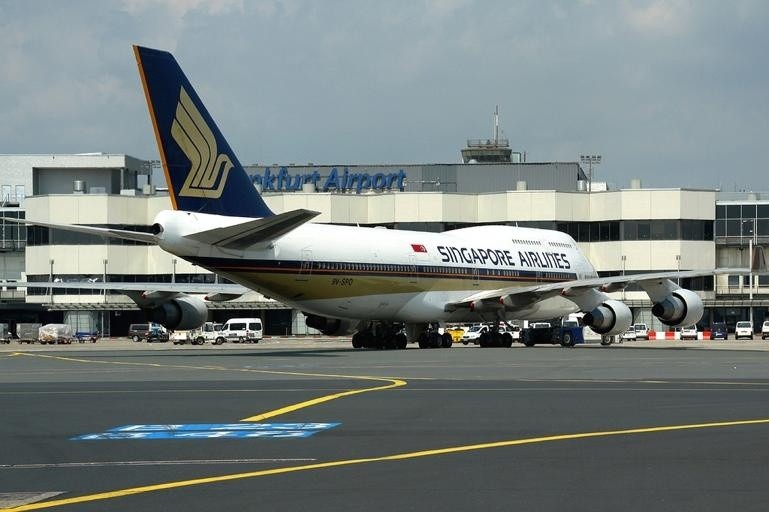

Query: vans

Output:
[633,322,649,340]
[734,321,753,339]
[679,323,698,339]
[710,322,728,340]
[461,318,580,345]
[128,316,263,345]
[761,320,769,339]
[621,325,636,341]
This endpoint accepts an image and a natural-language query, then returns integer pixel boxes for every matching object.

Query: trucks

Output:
[0,323,97,344]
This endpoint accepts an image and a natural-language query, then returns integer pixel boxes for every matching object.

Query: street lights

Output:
[620,255,627,299]
[102,259,109,302]
[47,259,54,304]
[170,259,177,283]
[577,154,605,193]
[675,255,681,285]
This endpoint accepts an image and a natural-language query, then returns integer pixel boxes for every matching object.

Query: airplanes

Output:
[0,43,704,351]
